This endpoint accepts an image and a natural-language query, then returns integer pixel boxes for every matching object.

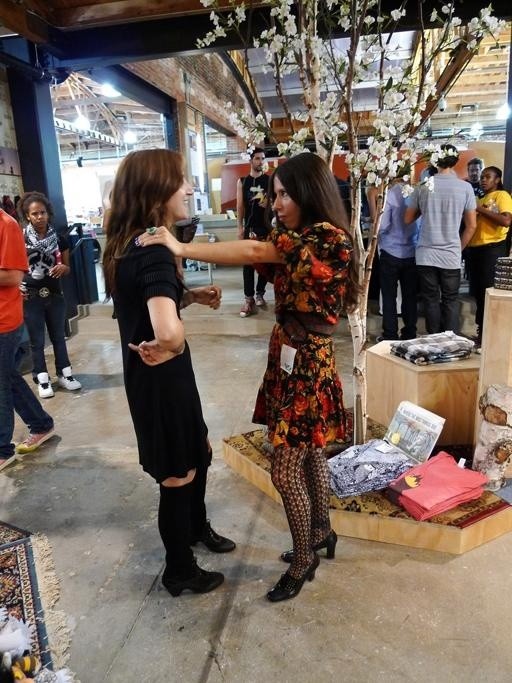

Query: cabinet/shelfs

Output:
[475,286,512,481]
[367,340,481,445]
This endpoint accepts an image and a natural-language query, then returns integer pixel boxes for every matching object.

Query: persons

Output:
[134,152,363,604]
[236,148,276,317]
[465,158,484,198]
[16,191,82,398]
[0,206,56,471]
[101,149,236,597]
[466,166,512,345]
[404,144,477,334]
[376,159,423,342]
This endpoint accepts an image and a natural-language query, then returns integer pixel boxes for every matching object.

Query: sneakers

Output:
[0,452,17,471]
[240,296,255,318]
[36,371,54,399]
[255,293,265,307]
[57,365,82,391]
[16,422,56,454]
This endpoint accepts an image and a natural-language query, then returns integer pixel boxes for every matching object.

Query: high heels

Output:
[189,519,236,553]
[280,529,338,562]
[161,557,225,598]
[266,551,322,602]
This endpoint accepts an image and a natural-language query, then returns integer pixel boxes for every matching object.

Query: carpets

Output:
[0,524,79,683]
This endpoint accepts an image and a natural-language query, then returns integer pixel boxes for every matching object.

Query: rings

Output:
[146,226,157,234]
